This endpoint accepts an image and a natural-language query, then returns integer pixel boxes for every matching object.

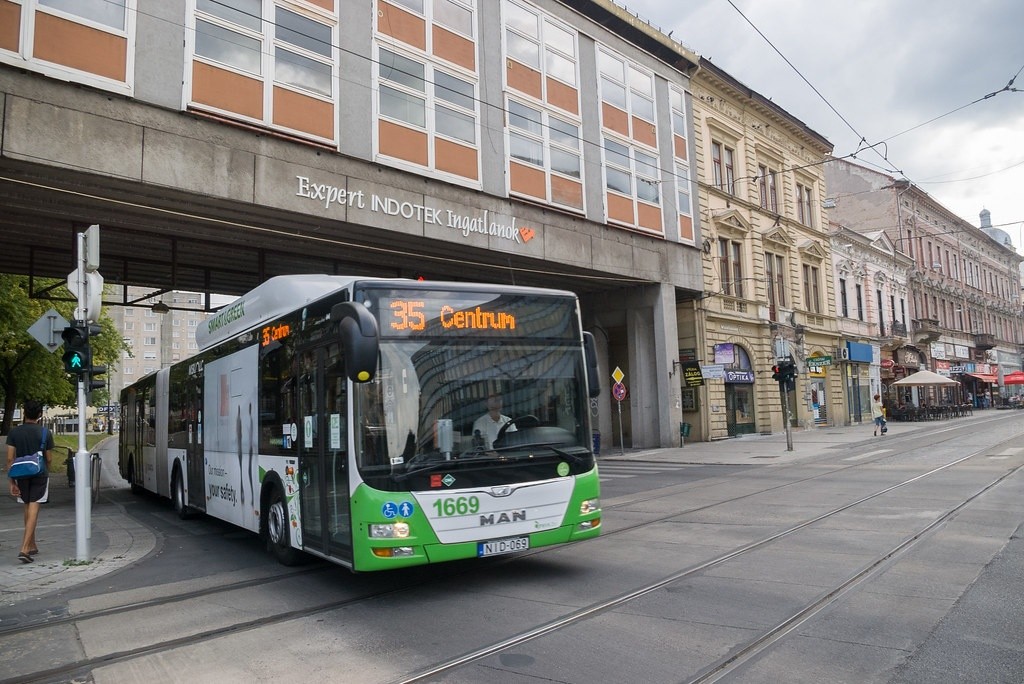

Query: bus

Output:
[117,273,604,574]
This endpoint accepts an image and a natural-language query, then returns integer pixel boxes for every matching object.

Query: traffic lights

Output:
[62,326,89,374]
[784,360,798,390]
[86,323,106,391]
[771,363,784,382]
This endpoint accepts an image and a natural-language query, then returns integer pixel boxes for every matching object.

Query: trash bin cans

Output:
[681,423,692,437]
[66,451,77,486]
[91,453,101,504]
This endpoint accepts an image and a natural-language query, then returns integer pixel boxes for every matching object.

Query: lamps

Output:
[1012,294,1019,299]
[932,263,941,268]
[794,326,805,344]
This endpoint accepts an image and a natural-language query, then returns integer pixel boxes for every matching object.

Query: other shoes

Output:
[29,548,38,554]
[18,552,34,563]
[881,434,884,435]
[874,431,876,436]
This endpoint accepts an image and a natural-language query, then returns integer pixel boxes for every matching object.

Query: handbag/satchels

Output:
[881,427,887,432]
[8,452,45,478]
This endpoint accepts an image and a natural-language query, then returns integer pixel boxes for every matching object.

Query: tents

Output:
[994,370,1024,384]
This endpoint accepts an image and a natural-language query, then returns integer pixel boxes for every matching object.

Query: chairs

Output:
[463,398,489,436]
[890,404,974,422]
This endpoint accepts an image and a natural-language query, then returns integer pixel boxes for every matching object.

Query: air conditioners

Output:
[836,347,849,361]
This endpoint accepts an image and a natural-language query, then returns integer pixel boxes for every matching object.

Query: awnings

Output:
[965,372,997,383]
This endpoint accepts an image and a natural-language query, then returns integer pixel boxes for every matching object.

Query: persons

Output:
[898,399,908,415]
[1004,392,1024,408]
[966,390,973,405]
[977,388,984,410]
[985,389,990,410]
[473,395,517,454]
[5,400,56,563]
[871,394,886,436]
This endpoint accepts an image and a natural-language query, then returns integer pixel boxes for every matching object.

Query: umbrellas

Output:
[888,370,960,418]
